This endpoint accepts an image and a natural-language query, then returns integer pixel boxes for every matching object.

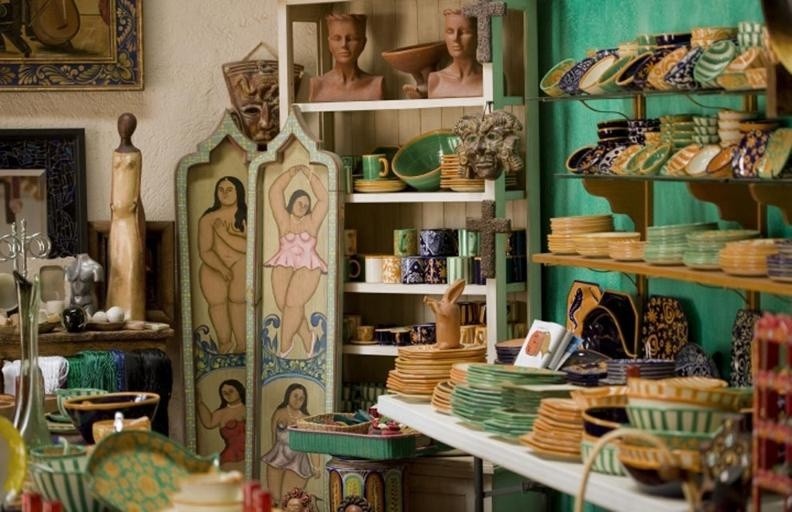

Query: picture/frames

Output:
[1,0,146,93]
[174,108,257,474]
[243,105,347,512]
[0,130,90,258]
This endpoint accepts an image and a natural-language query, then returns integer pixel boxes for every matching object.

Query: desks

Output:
[1,321,174,358]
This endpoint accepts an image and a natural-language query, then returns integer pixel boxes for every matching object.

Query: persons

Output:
[196,379,246,471]
[306,13,385,102]
[262,163,328,359]
[196,175,248,355]
[259,382,320,504]
[427,10,483,99]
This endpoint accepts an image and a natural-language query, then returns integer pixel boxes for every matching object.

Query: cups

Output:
[361,153,390,181]
[341,222,528,349]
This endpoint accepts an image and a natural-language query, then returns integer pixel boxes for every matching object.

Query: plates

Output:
[1,314,63,336]
[384,280,769,497]
[370,143,402,177]
[543,212,792,284]
[84,430,222,511]
[87,320,127,332]
[44,412,77,435]
[351,177,410,194]
[438,152,521,192]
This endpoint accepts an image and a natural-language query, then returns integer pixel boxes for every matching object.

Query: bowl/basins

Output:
[339,153,360,172]
[537,20,792,182]
[54,386,109,418]
[379,40,446,73]
[391,128,467,191]
[64,391,160,444]
[27,442,106,512]
[759,1,792,75]
